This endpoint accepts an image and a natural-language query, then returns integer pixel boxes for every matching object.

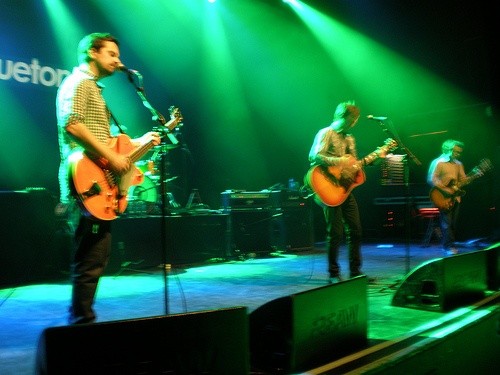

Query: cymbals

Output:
[147,144,178,151]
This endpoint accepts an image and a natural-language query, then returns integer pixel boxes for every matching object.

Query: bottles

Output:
[288,178,295,190]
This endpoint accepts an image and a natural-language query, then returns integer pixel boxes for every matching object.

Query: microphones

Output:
[116,63,140,76]
[368,115,387,121]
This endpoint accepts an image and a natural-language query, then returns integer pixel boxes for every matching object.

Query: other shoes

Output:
[351,273,375,281]
[446,248,458,256]
[330,276,341,283]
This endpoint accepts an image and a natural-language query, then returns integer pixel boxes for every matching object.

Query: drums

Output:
[134,160,153,174]
[126,175,158,203]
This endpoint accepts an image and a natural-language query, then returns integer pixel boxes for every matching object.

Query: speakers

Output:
[477,241,500,291]
[391,248,495,314]
[250,274,368,375]
[35,305,252,375]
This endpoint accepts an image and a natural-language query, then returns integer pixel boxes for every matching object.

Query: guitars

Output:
[66,106,184,221]
[430,157,492,208]
[304,138,399,208]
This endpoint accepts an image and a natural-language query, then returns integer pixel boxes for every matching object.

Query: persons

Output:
[308,101,387,284]
[56,33,160,325]
[427,139,484,254]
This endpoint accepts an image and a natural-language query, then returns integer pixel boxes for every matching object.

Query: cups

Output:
[220,189,235,209]
